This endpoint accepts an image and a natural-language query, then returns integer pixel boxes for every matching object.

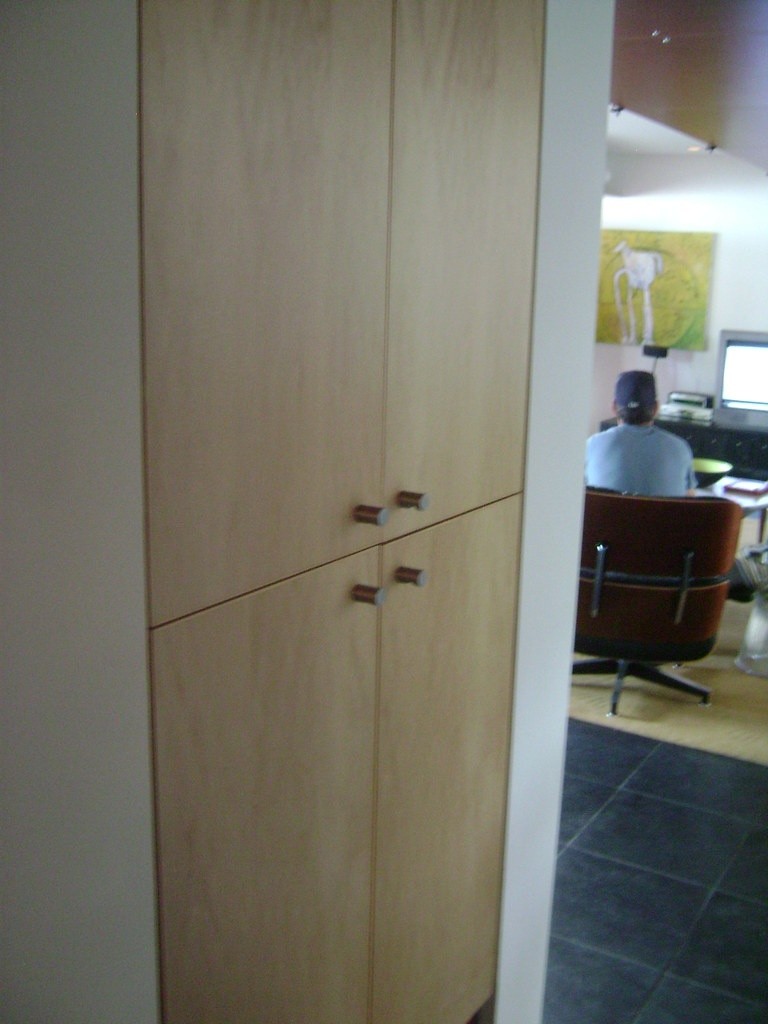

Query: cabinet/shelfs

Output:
[137,1,549,1024]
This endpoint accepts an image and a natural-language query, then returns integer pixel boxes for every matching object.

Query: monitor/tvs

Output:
[713,330,768,432]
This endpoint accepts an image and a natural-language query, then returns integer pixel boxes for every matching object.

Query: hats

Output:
[614,369,657,410]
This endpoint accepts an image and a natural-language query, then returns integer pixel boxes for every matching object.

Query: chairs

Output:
[570,484,742,718]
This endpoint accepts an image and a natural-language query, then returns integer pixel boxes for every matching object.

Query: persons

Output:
[587,371,698,497]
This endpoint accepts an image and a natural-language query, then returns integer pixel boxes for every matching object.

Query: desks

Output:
[600,403,768,481]
[690,475,768,549]
